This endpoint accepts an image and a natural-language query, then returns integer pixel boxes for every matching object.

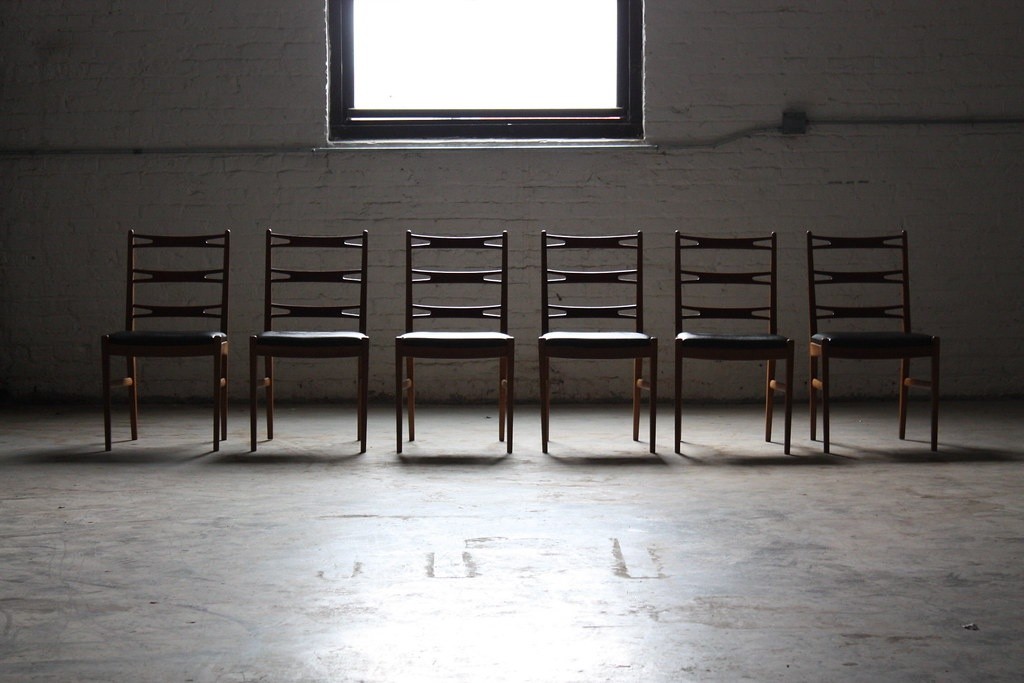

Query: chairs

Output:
[674,229,796,456]
[392,228,515,454]
[99,228,231,453]
[807,228,942,455]
[247,228,371,454]
[536,229,659,453]
